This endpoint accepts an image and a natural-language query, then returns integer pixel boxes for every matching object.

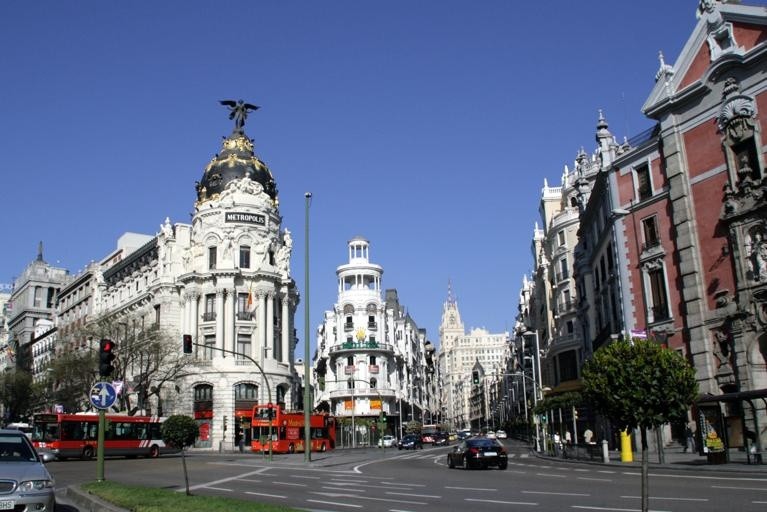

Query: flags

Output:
[5,332,17,364]
[246,283,258,313]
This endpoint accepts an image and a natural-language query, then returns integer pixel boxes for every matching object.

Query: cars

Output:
[0,427,58,512]
[376,428,509,451]
[446,437,508,470]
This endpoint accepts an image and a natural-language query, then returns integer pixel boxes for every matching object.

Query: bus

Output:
[28,410,185,463]
[248,403,338,455]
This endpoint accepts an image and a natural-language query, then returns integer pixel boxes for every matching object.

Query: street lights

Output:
[487,368,553,442]
[522,354,541,452]
[302,189,313,465]
[523,328,547,453]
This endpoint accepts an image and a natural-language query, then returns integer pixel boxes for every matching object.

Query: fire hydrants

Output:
[599,439,612,463]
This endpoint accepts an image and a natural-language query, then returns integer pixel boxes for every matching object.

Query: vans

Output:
[7,422,42,444]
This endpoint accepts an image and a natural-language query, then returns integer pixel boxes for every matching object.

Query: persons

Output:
[582,424,594,455]
[227,99,252,129]
[683,417,715,455]
[239,171,254,194]
[553,431,560,457]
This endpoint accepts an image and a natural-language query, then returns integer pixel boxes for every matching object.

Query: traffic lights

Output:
[268,403,273,418]
[184,335,193,353]
[573,410,578,421]
[473,371,480,385]
[98,338,116,376]
[541,411,548,424]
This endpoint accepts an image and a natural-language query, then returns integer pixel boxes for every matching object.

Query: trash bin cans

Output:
[554,442,564,457]
